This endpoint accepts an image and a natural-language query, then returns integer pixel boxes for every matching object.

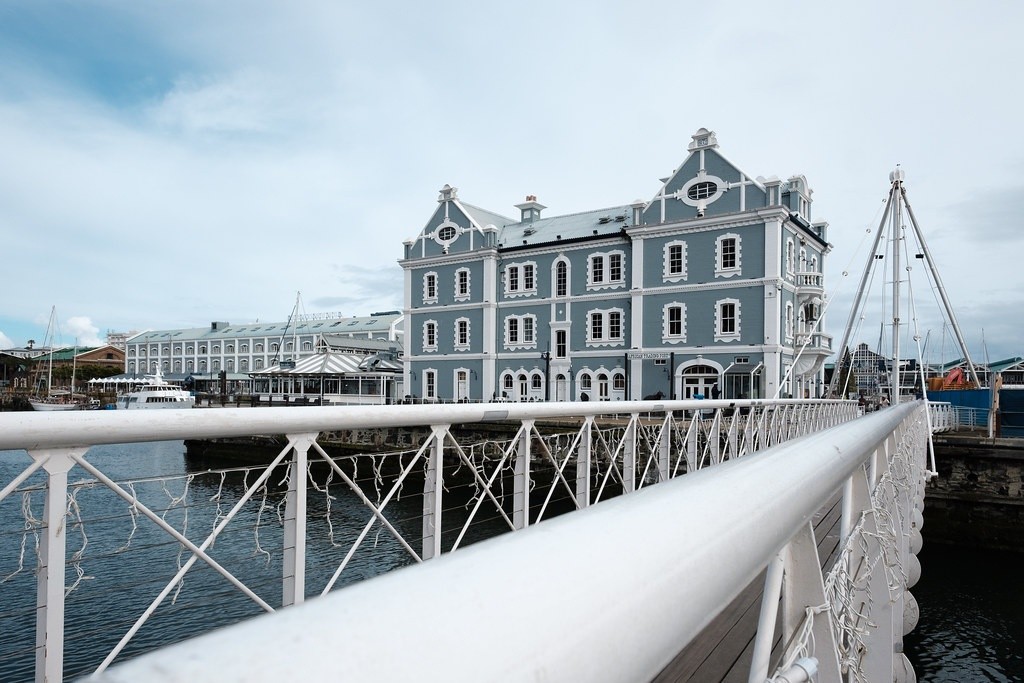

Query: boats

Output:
[112,384,195,410]
[26,305,100,411]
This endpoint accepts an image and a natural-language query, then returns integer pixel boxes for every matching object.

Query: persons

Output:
[878,395,891,407]
[851,393,868,406]
[820,392,826,398]
[710,382,721,413]
[391,392,590,405]
